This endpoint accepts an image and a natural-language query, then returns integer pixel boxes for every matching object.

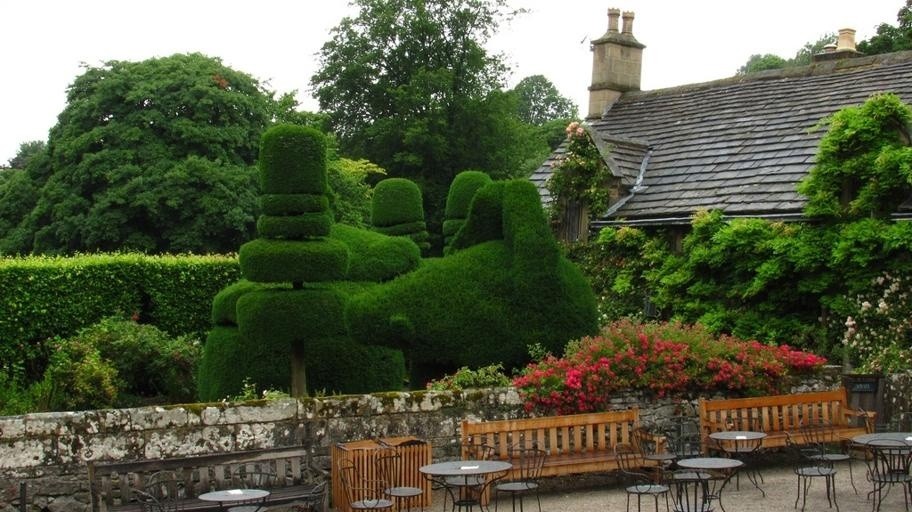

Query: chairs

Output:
[783,411,912,512]
[615,417,765,512]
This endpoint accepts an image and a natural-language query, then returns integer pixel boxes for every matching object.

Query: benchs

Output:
[85,440,332,512]
[698,386,878,469]
[460,405,667,506]
